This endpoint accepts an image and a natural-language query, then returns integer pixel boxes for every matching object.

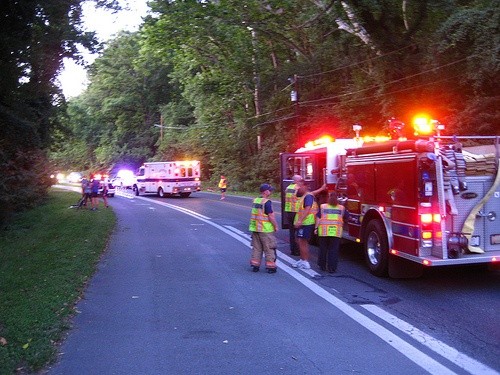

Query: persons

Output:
[248,184,279,273]
[101,176,109,208]
[285,175,326,256]
[314,192,356,273]
[77,175,100,210]
[218,174,228,200]
[291,179,315,269]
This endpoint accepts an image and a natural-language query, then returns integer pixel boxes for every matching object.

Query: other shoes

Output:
[317,264,325,270]
[252,266,259,272]
[220,197,225,200]
[292,250,298,255]
[268,267,276,272]
[329,271,336,275]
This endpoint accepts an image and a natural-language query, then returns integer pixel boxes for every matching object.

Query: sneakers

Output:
[291,259,310,269]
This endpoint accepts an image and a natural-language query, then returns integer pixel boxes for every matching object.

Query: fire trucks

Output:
[133,160,202,199]
[279,135,500,279]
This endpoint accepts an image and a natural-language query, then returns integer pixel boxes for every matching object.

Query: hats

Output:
[260,184,275,190]
[291,175,302,180]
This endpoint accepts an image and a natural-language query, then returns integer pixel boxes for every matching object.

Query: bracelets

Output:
[315,227,318,229]
